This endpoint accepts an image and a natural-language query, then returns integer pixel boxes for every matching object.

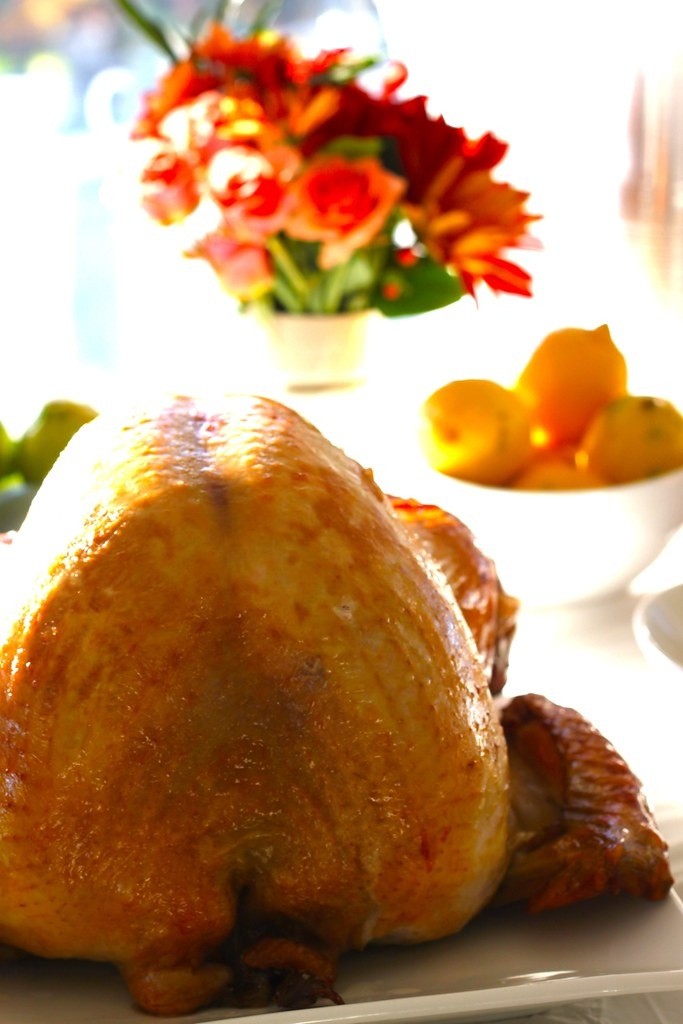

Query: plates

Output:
[0,886,683,1024]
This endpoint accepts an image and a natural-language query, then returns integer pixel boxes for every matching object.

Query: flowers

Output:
[108,0,542,322]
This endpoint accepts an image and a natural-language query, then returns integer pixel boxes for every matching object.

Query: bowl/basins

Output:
[425,469,683,598]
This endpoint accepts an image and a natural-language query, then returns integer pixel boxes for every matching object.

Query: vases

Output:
[255,311,369,385]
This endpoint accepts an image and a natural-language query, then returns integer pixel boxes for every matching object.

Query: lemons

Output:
[419,322,683,492]
[0,399,102,535]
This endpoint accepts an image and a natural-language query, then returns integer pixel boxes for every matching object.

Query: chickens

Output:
[0,393,673,1018]
